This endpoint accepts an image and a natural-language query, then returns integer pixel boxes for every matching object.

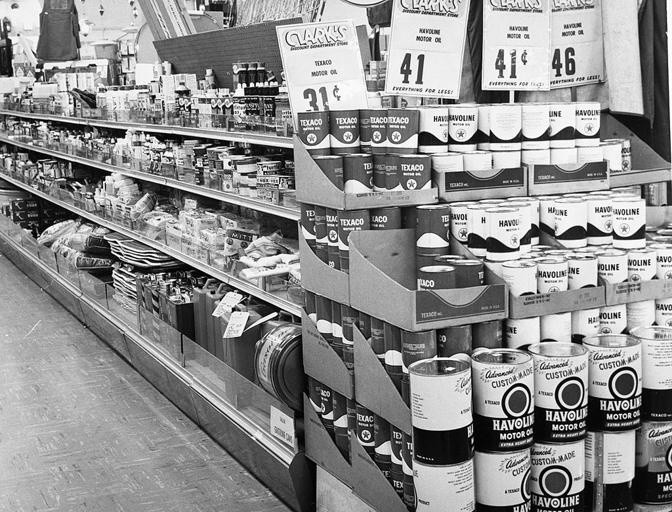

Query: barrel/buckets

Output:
[192,278,302,413]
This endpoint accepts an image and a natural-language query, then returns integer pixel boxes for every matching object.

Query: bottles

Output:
[96,60,294,137]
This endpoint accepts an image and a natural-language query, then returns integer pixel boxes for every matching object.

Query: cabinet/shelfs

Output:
[0,109,672,512]
